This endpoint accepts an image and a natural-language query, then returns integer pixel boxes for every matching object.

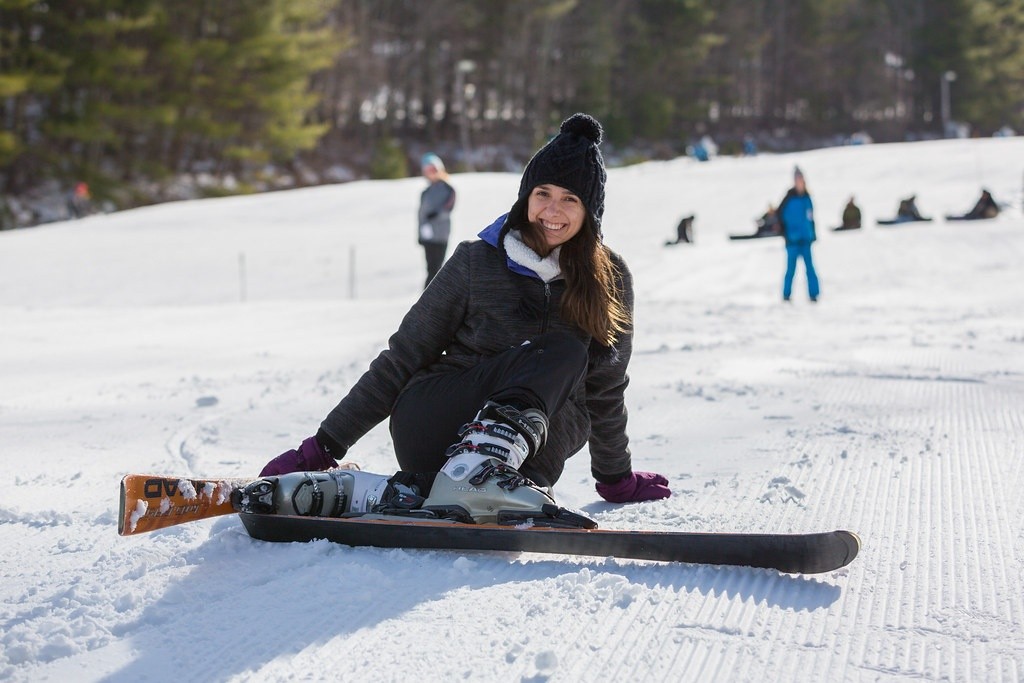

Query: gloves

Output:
[595,472,671,503]
[258,435,333,477]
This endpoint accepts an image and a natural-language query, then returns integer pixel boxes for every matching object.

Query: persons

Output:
[257,114,672,523]
[418,153,455,289]
[669,132,1003,244]
[776,166,818,303]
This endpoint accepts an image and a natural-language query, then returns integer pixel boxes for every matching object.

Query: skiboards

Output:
[116,471,861,576]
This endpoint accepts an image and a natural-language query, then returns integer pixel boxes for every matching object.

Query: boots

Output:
[420,401,557,525]
[274,471,392,518]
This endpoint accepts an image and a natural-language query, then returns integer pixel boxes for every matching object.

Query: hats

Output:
[518,113,607,228]
